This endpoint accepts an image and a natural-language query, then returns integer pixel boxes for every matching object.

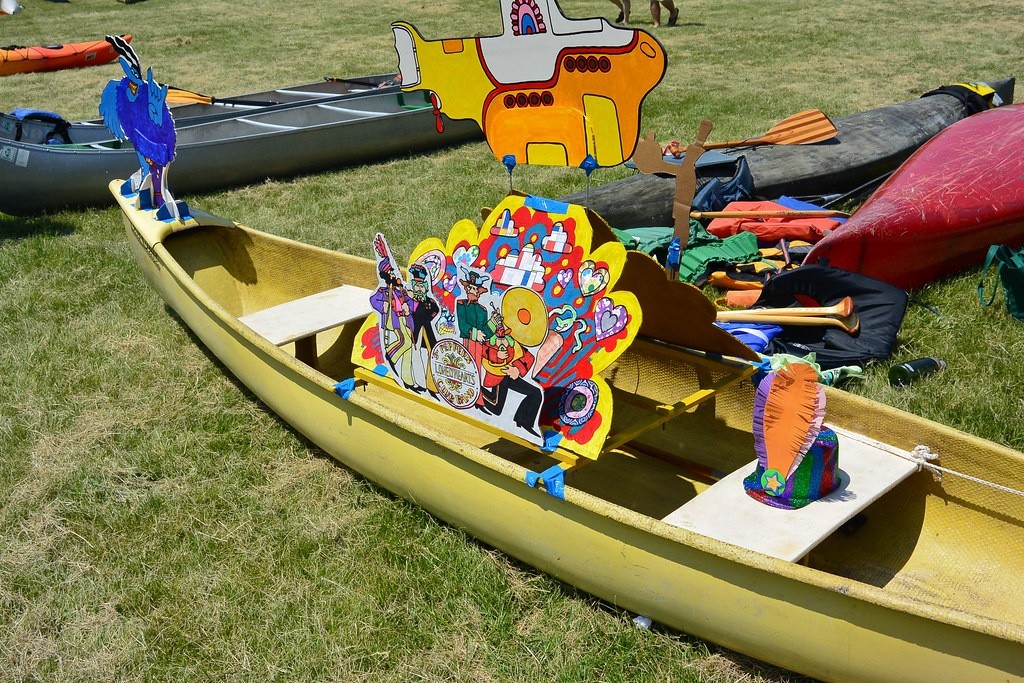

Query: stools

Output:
[657,421,926,567]
[238,282,379,370]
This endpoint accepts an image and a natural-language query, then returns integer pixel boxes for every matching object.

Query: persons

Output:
[650,0,679,26]
[609,0,631,25]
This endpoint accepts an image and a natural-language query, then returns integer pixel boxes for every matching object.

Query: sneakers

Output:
[616,11,631,23]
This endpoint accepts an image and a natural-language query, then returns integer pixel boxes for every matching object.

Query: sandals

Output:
[667,7,679,26]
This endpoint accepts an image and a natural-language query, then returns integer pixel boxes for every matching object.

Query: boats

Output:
[799,104,1024,295]
[548,72,1017,226]
[0,91,485,218]
[0,70,406,146]
[101,167,1024,682]
[0,34,137,76]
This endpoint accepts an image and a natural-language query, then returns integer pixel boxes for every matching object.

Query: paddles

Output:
[167,87,283,106]
[670,108,839,159]
